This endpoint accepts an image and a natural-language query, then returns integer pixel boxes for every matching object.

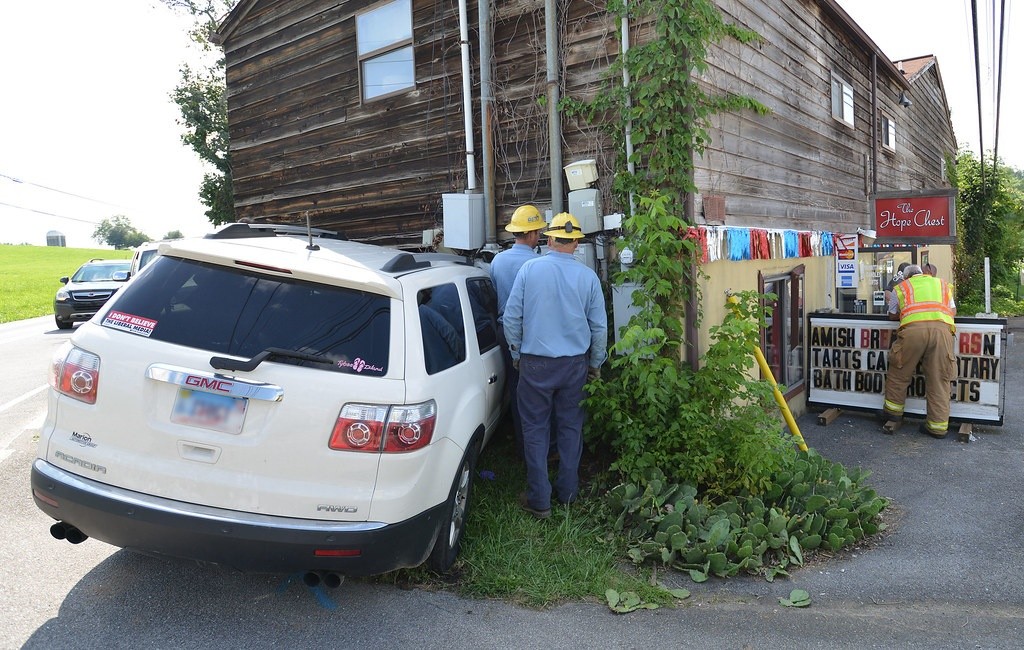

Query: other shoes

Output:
[875,410,903,422]
[918,424,948,439]
[547,451,560,464]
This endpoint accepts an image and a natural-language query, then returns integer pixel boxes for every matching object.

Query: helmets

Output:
[505,205,548,232]
[543,212,585,238]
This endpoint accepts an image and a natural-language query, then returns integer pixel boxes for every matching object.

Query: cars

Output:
[766,307,803,342]
[112,237,246,304]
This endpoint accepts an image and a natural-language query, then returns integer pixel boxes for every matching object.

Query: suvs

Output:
[53,258,134,329]
[30,221,512,589]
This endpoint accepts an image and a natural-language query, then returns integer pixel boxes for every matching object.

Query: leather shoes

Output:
[519,490,552,519]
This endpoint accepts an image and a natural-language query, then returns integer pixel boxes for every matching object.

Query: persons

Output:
[876,262,957,439]
[489,205,607,519]
[416,284,466,376]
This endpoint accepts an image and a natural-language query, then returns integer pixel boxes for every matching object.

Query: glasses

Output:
[926,263,930,269]
